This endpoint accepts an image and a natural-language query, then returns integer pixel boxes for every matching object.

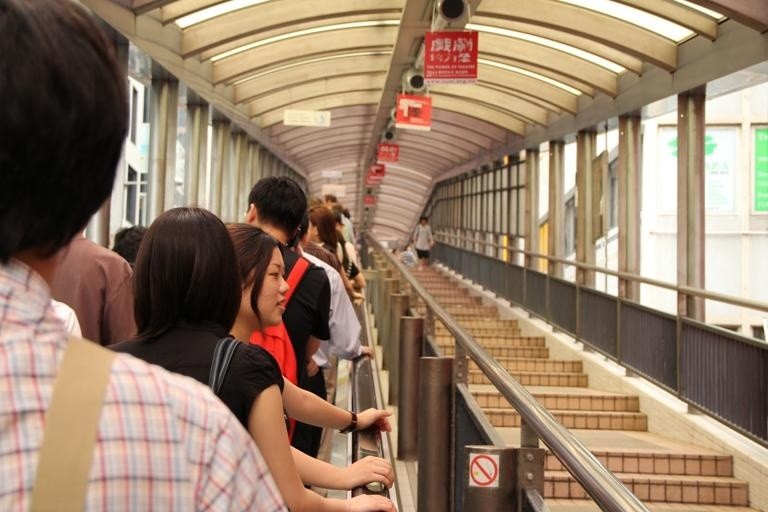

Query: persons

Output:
[243,175,332,455]
[412,216,433,268]
[304,193,367,300]
[0,1,286,512]
[299,213,376,488]
[224,222,395,492]
[107,205,397,511]
[112,224,148,268]
[53,228,139,346]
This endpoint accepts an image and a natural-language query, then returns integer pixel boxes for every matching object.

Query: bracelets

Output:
[339,412,357,435]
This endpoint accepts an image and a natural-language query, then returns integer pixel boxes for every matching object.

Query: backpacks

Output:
[249,256,309,445]
